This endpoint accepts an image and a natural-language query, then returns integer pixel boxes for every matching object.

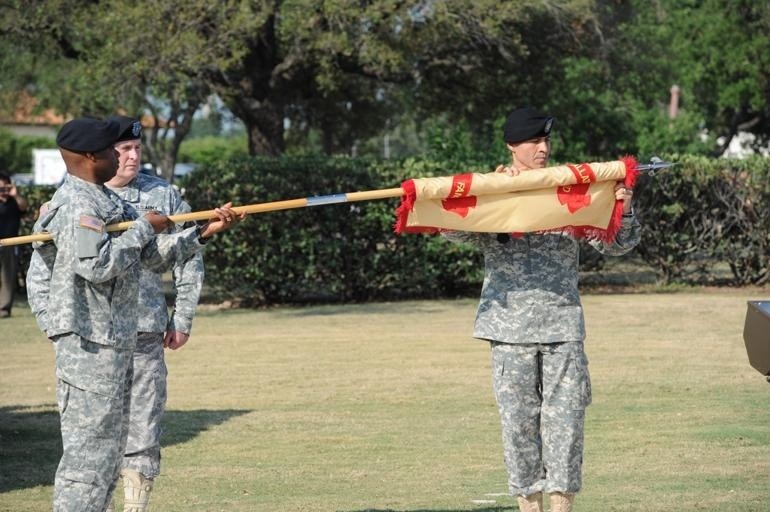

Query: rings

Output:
[226,217,232,221]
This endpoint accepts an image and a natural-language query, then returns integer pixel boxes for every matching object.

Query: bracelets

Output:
[197,228,207,244]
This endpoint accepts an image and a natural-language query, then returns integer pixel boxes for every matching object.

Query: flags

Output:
[396,150,639,241]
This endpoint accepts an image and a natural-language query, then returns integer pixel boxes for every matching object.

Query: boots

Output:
[517,492,544,512]
[119,469,155,512]
[549,493,575,512]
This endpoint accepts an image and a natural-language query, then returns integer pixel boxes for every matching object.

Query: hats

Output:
[55,115,142,152]
[503,107,554,142]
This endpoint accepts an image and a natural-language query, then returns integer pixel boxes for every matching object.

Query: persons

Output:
[439,106,641,512]
[25,115,205,511]
[1,172,29,317]
[43,118,247,512]
